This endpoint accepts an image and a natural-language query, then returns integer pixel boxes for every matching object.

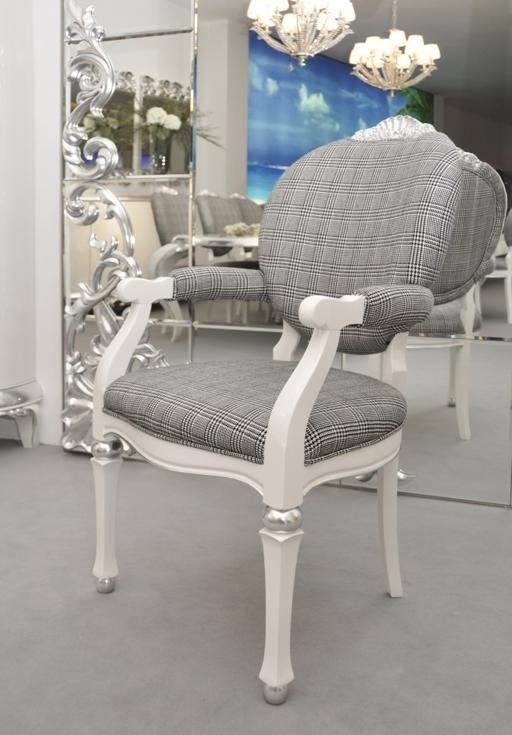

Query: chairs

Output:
[87,107,465,708]
